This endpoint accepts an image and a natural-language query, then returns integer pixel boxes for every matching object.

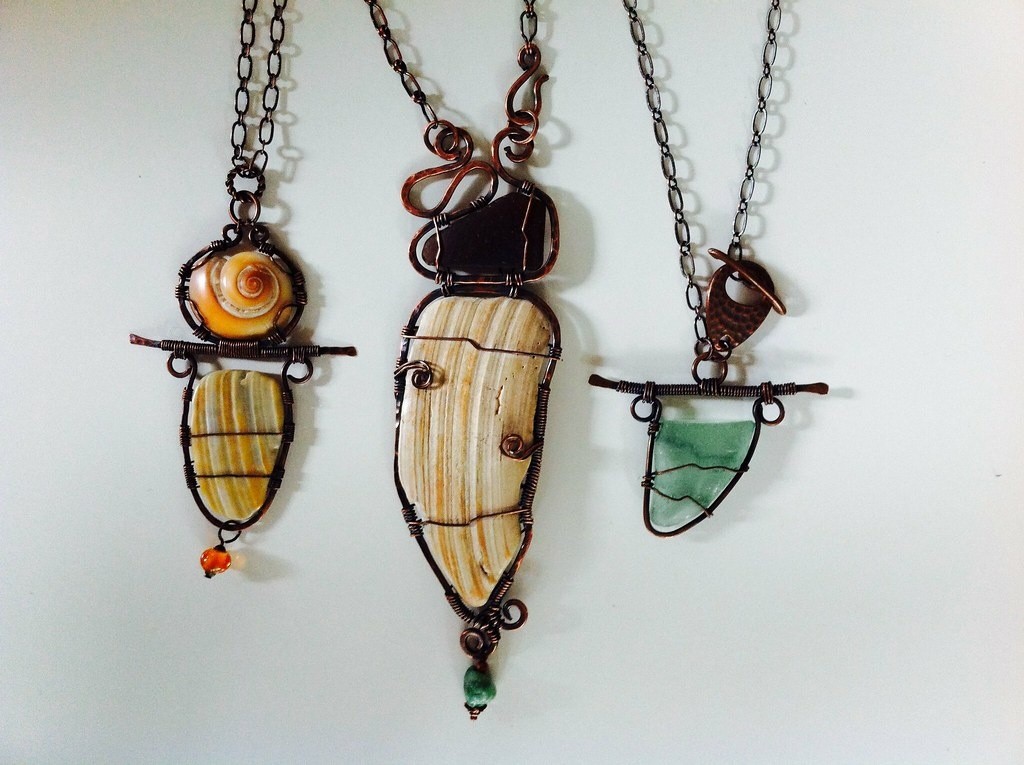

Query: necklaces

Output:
[588,0,829,537]
[364,1,561,721]
[130,1,357,579]
